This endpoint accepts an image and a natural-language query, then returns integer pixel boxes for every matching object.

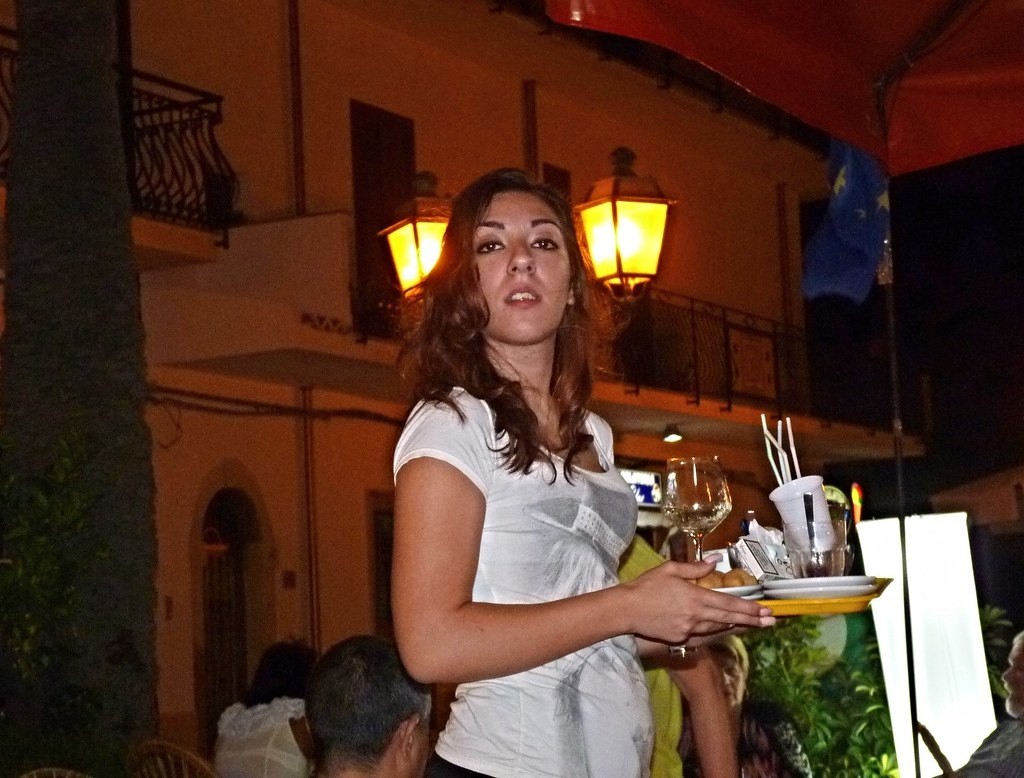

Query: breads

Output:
[691,568,758,588]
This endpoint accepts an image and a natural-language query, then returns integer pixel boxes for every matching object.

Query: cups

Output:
[769,475,836,550]
[781,522,847,578]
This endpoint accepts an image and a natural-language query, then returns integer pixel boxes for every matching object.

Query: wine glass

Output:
[661,456,733,562]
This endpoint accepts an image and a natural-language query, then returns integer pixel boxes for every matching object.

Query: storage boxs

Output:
[736,538,794,581]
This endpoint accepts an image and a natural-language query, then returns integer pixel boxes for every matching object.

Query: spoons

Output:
[802,493,829,577]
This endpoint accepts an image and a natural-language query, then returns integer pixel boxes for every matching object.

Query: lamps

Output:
[377,171,452,307]
[574,147,680,397]
[662,423,683,443]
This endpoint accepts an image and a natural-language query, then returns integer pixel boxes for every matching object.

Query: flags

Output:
[801,140,893,310]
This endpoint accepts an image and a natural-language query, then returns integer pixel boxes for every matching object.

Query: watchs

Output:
[671,642,700,662]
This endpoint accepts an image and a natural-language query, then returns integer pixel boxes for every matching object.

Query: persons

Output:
[208,637,316,778]
[944,633,1024,778]
[389,163,800,778]
[298,632,437,777]
[619,529,818,778]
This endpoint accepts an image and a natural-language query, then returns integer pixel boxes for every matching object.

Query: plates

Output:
[707,584,763,599]
[763,576,877,599]
